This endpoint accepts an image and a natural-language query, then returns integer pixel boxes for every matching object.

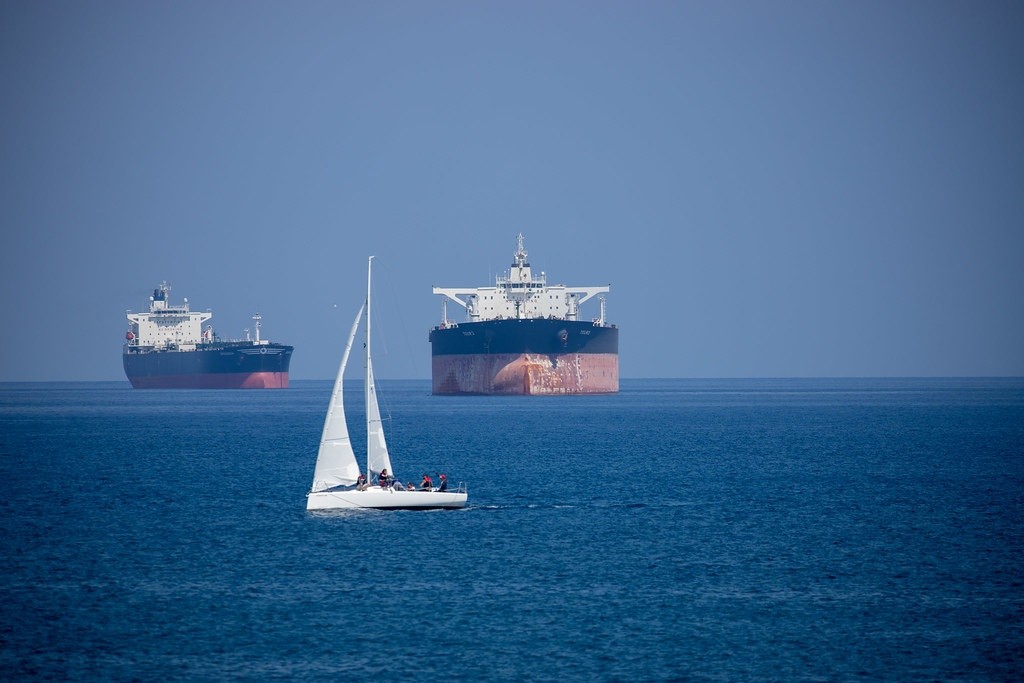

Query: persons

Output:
[437,475,447,492]
[356,475,367,491]
[379,469,388,487]
[407,482,415,491]
[420,474,433,492]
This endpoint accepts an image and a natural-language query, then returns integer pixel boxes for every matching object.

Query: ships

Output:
[428,233,619,395]
[123,280,294,389]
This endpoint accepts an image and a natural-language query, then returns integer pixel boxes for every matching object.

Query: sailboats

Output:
[306,256,468,507]
